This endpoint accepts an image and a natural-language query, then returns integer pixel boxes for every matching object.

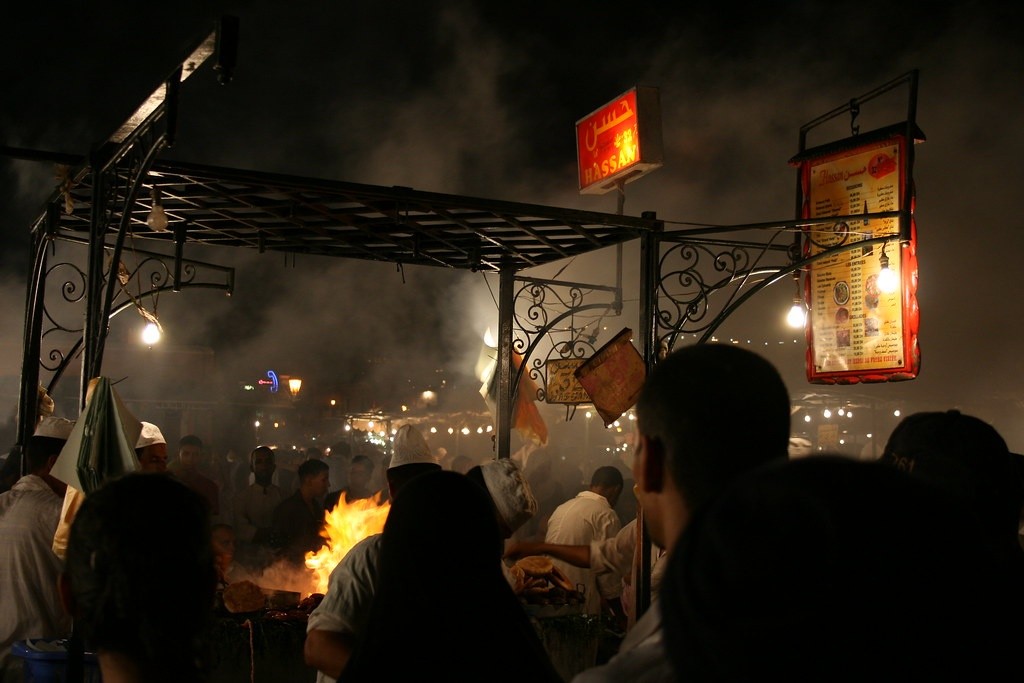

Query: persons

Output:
[0,321,1022,683]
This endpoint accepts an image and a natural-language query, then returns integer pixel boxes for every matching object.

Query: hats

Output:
[388,423,442,467]
[881,409,1011,482]
[33,416,77,441]
[481,457,537,537]
[134,421,167,450]
